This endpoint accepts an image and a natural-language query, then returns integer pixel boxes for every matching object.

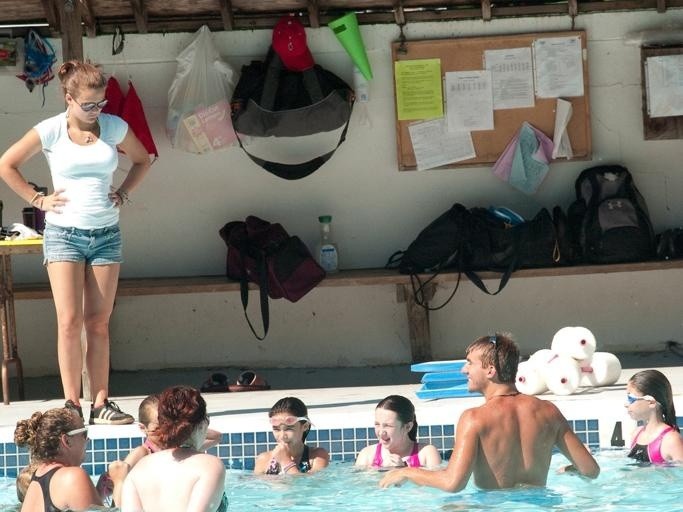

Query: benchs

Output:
[12,257,683,366]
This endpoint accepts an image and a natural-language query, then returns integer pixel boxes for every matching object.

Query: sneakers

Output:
[61,399,84,422]
[655,228,683,261]
[89,399,135,425]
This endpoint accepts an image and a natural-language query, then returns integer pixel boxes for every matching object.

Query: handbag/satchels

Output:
[408,202,560,296]
[218,215,326,304]
[230,56,356,181]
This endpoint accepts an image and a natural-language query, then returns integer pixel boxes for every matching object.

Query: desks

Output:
[1,234,44,407]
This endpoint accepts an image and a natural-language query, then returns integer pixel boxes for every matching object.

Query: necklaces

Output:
[487,391,522,401]
[66,112,95,143]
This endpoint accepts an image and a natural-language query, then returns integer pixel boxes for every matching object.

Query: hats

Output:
[25,29,56,76]
[272,16,314,72]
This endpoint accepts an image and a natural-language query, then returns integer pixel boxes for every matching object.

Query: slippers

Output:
[202,370,271,392]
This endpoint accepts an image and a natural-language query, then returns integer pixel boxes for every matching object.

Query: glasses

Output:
[24,64,35,93]
[65,426,89,441]
[112,26,125,56]
[272,415,308,425]
[70,95,109,112]
[489,338,501,380]
[626,394,653,402]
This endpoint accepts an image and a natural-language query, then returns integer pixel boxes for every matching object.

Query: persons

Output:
[623,368,683,467]
[252,396,330,477]
[355,394,443,469]
[119,385,230,512]
[0,59,152,425]
[14,408,130,512]
[378,332,601,494]
[107,392,222,509]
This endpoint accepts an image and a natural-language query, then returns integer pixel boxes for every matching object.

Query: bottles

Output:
[28,180,47,230]
[316,215,338,275]
[22,207,34,226]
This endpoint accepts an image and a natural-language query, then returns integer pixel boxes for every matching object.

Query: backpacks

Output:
[568,165,656,264]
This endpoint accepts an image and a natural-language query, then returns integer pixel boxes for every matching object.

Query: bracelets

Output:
[282,461,296,473]
[115,188,130,207]
[30,191,44,209]
